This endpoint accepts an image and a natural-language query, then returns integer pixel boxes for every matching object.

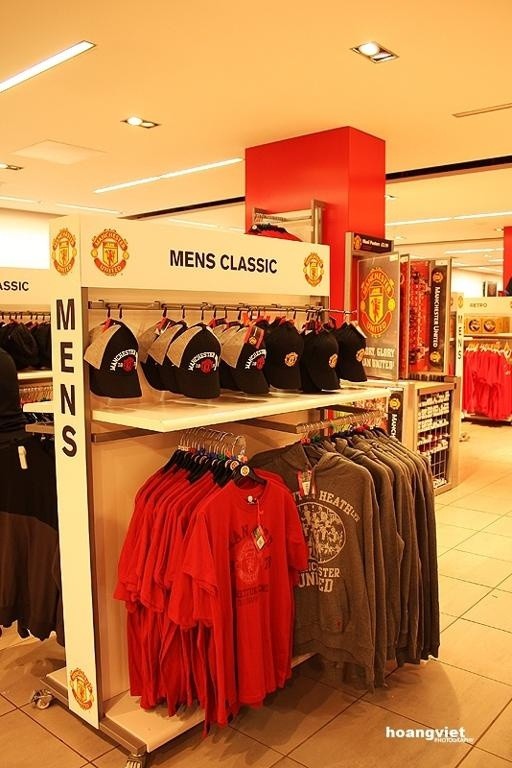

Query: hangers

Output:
[163,429,267,489]
[248,208,287,233]
[17,385,54,422]
[296,410,392,458]
[31,422,54,442]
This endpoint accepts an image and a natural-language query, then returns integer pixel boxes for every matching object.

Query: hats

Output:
[301,321,324,337]
[262,319,305,390]
[242,311,286,329]
[220,324,270,395]
[82,321,143,399]
[145,320,190,395]
[87,318,121,397]
[328,316,368,382]
[137,317,177,391]
[208,318,228,337]
[166,321,222,400]
[301,327,341,390]
[218,320,244,392]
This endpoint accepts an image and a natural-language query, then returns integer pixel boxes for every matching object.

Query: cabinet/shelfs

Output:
[0,266,49,651]
[30,216,390,768]
